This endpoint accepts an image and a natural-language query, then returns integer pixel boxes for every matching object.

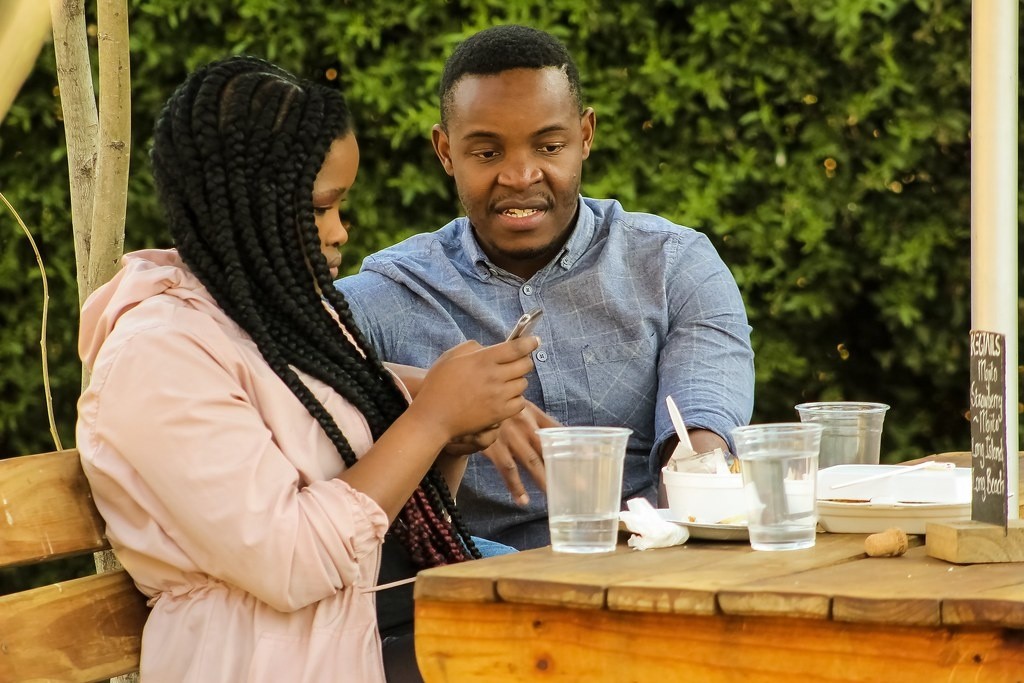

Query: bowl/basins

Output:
[662,465,743,522]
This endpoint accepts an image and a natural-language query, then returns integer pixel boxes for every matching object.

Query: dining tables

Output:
[405,449,1024,682]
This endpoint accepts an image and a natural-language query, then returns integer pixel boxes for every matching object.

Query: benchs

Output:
[1,447,153,683]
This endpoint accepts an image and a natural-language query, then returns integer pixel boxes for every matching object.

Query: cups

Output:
[795,402,890,469]
[732,423,824,550]
[536,426,633,553]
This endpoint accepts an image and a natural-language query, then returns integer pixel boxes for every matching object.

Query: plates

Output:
[618,509,816,541]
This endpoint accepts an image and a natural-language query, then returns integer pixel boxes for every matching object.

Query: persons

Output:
[320,25,755,553]
[75,56,540,683]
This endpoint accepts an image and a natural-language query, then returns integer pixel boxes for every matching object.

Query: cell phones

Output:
[506,307,543,342]
